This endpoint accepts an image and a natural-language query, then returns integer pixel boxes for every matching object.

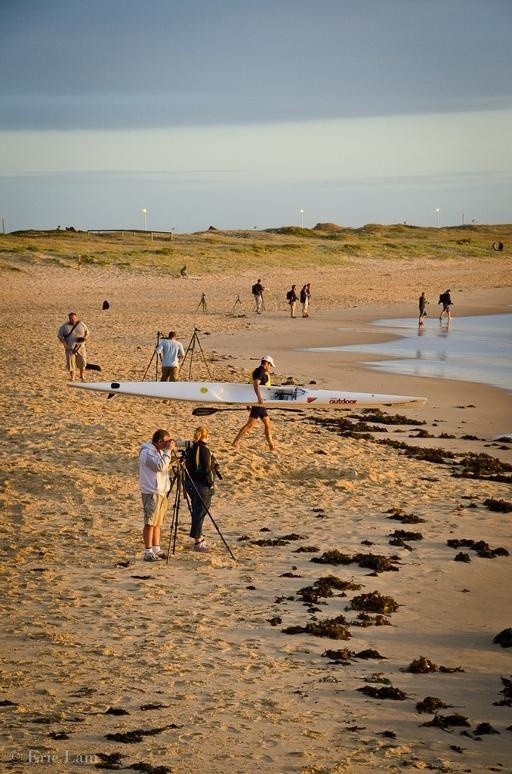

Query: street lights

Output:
[435,207,441,228]
[142,207,147,231]
[299,209,305,230]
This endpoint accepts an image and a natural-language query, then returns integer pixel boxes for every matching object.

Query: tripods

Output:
[178,331,214,380]
[195,291,209,315]
[229,293,245,315]
[142,334,169,382]
[254,287,267,312]
[167,449,236,567]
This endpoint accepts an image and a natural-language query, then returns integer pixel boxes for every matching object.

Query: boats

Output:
[66,382,426,409]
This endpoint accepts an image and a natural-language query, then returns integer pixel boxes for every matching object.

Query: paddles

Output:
[192,408,303,416]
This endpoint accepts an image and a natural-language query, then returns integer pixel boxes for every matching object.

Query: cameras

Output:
[157,331,162,335]
[195,327,201,332]
[77,337,85,343]
[426,301,430,304]
[170,439,194,448]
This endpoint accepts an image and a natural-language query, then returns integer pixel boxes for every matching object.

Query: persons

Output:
[58,313,90,381]
[252,279,264,314]
[231,356,276,451]
[440,290,453,320]
[139,429,175,561]
[287,284,311,318]
[180,427,215,549]
[419,292,427,324]
[157,331,185,381]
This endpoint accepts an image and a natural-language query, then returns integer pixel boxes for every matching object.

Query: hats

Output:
[261,355,276,368]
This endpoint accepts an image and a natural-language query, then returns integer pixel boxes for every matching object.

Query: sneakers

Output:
[194,540,216,553]
[156,551,168,558]
[144,552,161,561]
